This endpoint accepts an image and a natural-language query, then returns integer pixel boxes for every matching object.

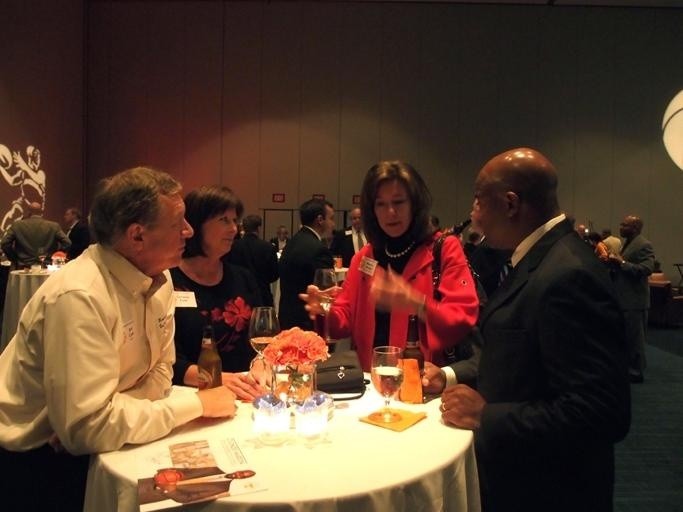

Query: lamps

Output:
[660,88,683,170]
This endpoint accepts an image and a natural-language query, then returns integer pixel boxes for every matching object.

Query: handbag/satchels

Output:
[313,351,370,401]
[431,232,491,363]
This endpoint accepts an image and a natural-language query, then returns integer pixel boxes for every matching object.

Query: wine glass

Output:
[247,306,280,408]
[368,344,401,423]
[35,247,47,272]
[313,268,337,344]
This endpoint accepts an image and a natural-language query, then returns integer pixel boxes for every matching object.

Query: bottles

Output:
[399,313,425,405]
[196,323,222,390]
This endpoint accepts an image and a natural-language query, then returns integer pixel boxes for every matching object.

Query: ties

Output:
[495,260,512,287]
[356,230,363,253]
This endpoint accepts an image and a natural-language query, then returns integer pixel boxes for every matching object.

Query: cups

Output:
[333,256,342,268]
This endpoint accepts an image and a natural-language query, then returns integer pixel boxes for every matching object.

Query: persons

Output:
[1,145,658,511]
